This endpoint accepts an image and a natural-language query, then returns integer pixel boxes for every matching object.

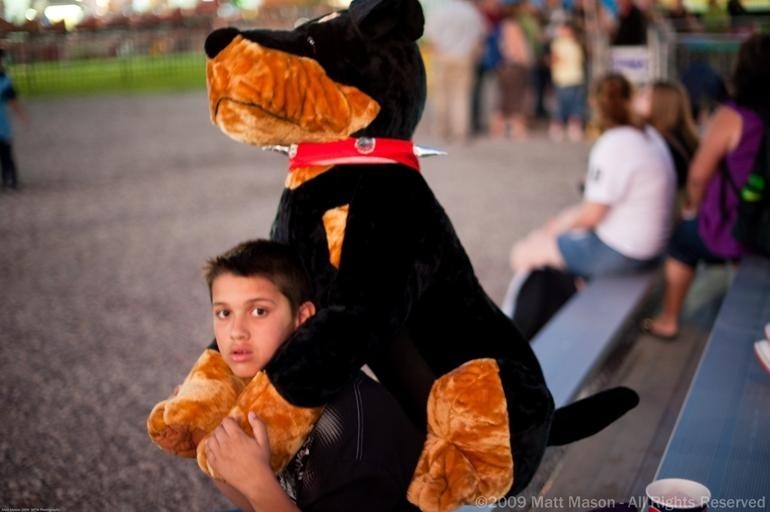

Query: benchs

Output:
[426,265,657,510]
[643,249,770,511]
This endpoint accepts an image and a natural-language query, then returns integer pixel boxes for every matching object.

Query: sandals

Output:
[639,316,678,340]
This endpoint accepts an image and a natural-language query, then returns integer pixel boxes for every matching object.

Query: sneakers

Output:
[754,322,770,375]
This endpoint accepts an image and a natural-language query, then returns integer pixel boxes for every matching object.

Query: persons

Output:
[426,0,769,377]
[147,0,640,512]
[0,50,35,188]
[201,241,427,511]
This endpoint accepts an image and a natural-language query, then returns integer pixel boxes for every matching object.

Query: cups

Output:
[644,478,711,512]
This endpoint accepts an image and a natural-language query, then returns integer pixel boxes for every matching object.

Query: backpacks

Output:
[720,103,769,257]
